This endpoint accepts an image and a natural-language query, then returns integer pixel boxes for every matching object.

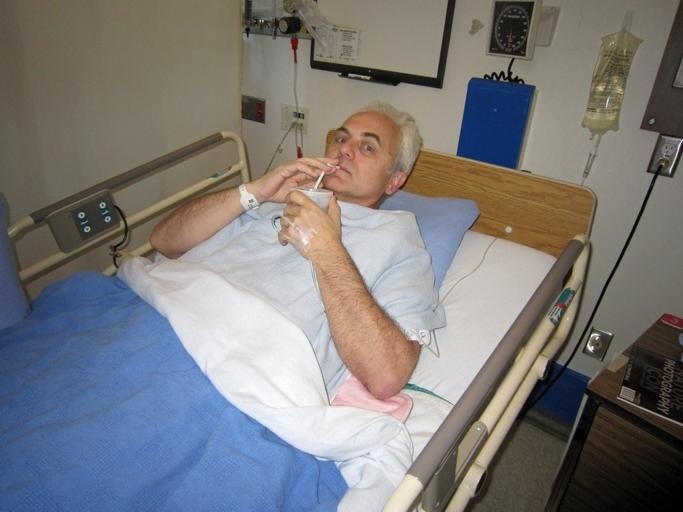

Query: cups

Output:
[289,186,333,213]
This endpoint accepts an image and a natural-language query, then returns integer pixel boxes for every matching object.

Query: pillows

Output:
[378,188,482,289]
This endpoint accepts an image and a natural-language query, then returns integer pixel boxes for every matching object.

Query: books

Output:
[617,344,682,425]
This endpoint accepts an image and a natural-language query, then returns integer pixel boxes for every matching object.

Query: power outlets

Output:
[649,135,683,178]
[582,326,614,361]
[281,102,310,135]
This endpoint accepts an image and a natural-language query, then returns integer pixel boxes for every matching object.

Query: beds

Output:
[0,131,596,512]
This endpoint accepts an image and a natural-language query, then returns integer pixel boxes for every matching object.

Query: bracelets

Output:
[238,183,259,212]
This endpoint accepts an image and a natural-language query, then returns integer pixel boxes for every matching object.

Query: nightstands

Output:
[545,314,682,511]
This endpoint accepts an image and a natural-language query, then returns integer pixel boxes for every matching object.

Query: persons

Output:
[147,97,445,407]
